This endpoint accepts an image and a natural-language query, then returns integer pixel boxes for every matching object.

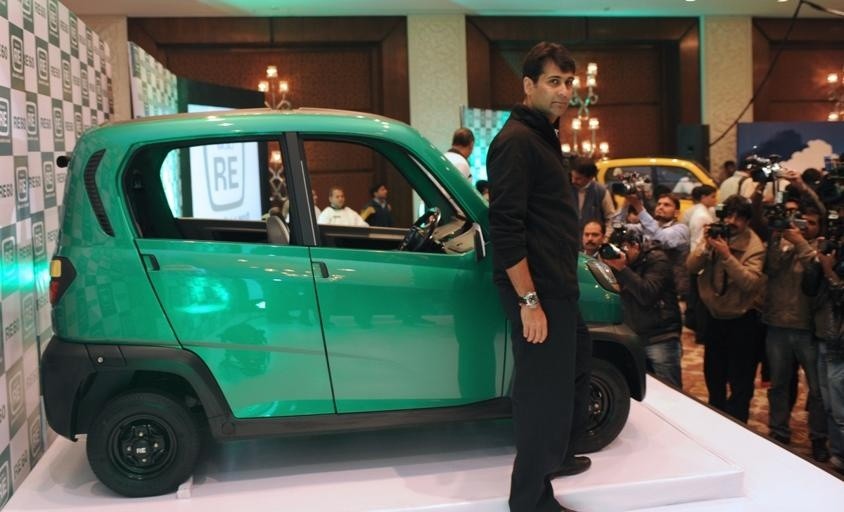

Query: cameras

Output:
[598,222,629,260]
[706,203,730,239]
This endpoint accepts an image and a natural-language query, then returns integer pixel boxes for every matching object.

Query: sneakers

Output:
[770,432,844,475]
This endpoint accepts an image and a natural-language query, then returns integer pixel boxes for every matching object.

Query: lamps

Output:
[827,74,844,122]
[268,151,288,202]
[258,65,290,110]
[567,63,599,120]
[559,118,609,162]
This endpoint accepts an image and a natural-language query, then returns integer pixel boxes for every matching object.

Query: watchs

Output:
[517,291,538,306]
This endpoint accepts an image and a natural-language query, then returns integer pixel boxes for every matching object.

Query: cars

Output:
[17,102,663,502]
[580,150,729,234]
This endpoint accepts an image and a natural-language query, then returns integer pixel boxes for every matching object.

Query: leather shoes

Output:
[542,456,591,479]
[543,498,577,511]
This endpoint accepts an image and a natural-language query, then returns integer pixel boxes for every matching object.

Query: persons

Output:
[284,128,489,229]
[484,42,591,512]
[568,151,844,474]
